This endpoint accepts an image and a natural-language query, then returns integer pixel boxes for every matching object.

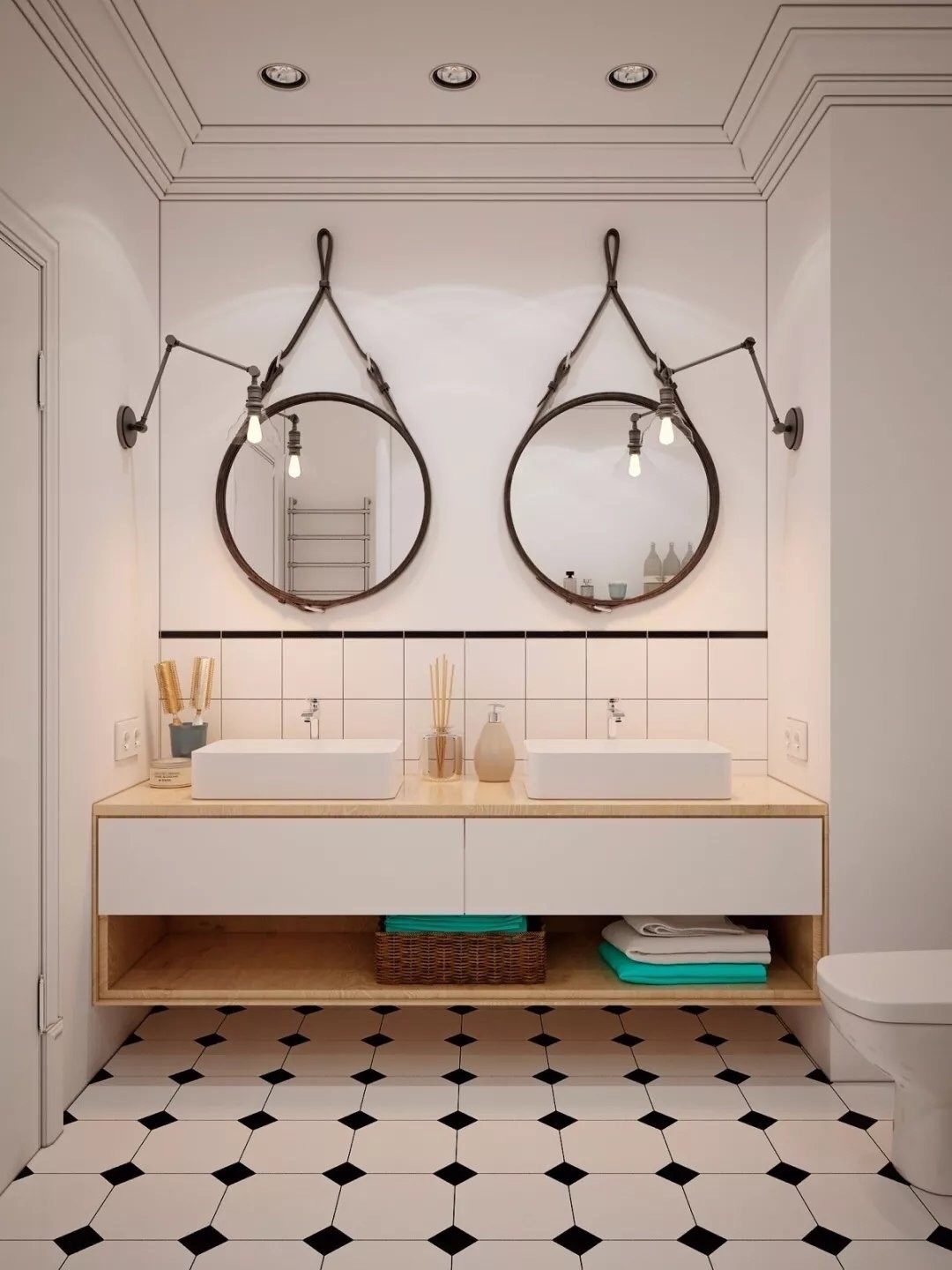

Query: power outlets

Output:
[785,718,809,761]
[115,717,144,761]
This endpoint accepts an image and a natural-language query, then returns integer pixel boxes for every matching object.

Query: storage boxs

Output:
[373,915,548,985]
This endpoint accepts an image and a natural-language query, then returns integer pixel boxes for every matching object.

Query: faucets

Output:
[300,698,321,739]
[606,697,625,739]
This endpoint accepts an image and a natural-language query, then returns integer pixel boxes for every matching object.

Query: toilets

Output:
[811,946,950,1197]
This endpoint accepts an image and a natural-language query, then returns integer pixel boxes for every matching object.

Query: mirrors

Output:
[504,392,720,607]
[216,392,432,609]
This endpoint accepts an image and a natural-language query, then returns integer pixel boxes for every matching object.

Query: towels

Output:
[599,915,774,986]
[383,914,528,934]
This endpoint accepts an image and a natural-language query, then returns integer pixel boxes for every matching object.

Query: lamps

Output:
[116,335,264,449]
[627,410,655,478]
[277,412,302,478]
[659,337,804,451]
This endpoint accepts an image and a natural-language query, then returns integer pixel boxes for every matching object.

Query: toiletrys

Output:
[472,702,517,782]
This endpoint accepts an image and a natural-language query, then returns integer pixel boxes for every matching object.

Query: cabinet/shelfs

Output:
[92,814,830,1008]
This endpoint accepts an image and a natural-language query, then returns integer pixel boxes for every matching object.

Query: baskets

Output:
[375,915,548,984]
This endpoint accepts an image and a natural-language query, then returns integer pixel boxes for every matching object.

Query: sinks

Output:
[521,738,732,802]
[189,737,407,800]
